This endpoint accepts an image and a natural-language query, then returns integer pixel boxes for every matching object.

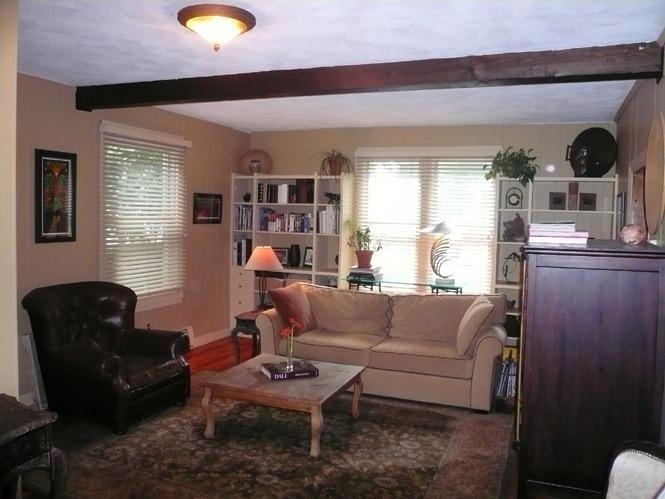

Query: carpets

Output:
[10,369,513,498]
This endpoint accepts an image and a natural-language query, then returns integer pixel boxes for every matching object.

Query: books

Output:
[346,270,383,282]
[260,360,319,380]
[317,206,340,233]
[525,222,589,248]
[258,180,313,202]
[506,336,520,346]
[233,206,252,230]
[233,238,251,266]
[258,209,314,232]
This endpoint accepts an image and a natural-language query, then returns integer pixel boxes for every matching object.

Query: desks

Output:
[1,394,57,498]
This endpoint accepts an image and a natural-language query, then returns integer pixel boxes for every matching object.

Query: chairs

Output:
[607,439,664,499]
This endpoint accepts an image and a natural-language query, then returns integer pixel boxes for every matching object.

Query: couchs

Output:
[22,281,190,434]
[256,283,506,413]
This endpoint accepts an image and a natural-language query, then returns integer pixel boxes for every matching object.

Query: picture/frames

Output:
[303,246,313,267]
[193,193,222,223]
[34,149,76,243]
[272,248,289,265]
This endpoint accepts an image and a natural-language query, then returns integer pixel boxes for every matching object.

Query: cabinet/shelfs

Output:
[492,177,617,347]
[231,173,341,327]
[516,239,664,499]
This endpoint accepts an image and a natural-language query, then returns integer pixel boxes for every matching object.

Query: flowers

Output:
[279,318,303,338]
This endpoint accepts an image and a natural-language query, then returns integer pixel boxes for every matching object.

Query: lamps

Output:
[243,246,283,313]
[176,7,253,54]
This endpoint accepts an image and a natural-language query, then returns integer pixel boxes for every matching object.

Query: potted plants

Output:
[311,150,352,184]
[345,219,384,269]
[485,147,538,187]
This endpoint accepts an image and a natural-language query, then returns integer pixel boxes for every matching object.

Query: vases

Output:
[286,335,293,372]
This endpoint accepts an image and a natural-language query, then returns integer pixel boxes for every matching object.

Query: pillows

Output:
[268,283,318,336]
[457,297,495,359]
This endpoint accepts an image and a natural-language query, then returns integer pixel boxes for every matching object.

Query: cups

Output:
[275,328,294,374]
